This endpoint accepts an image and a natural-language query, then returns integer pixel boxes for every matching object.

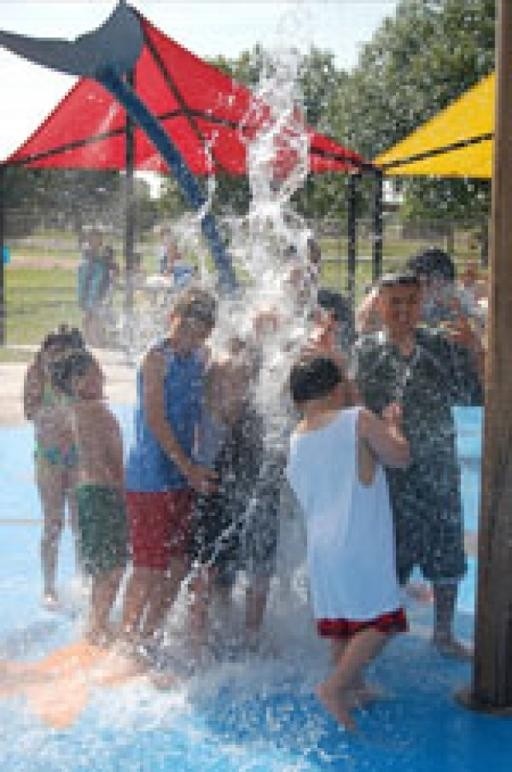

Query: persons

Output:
[132,254,148,291]
[157,225,199,286]
[22,320,88,611]
[283,354,411,730]
[50,348,128,646]
[101,246,117,333]
[346,271,486,660]
[355,248,457,334]
[454,267,479,316]
[209,288,356,655]
[180,309,285,665]
[74,229,107,345]
[264,240,324,615]
[118,287,219,657]
